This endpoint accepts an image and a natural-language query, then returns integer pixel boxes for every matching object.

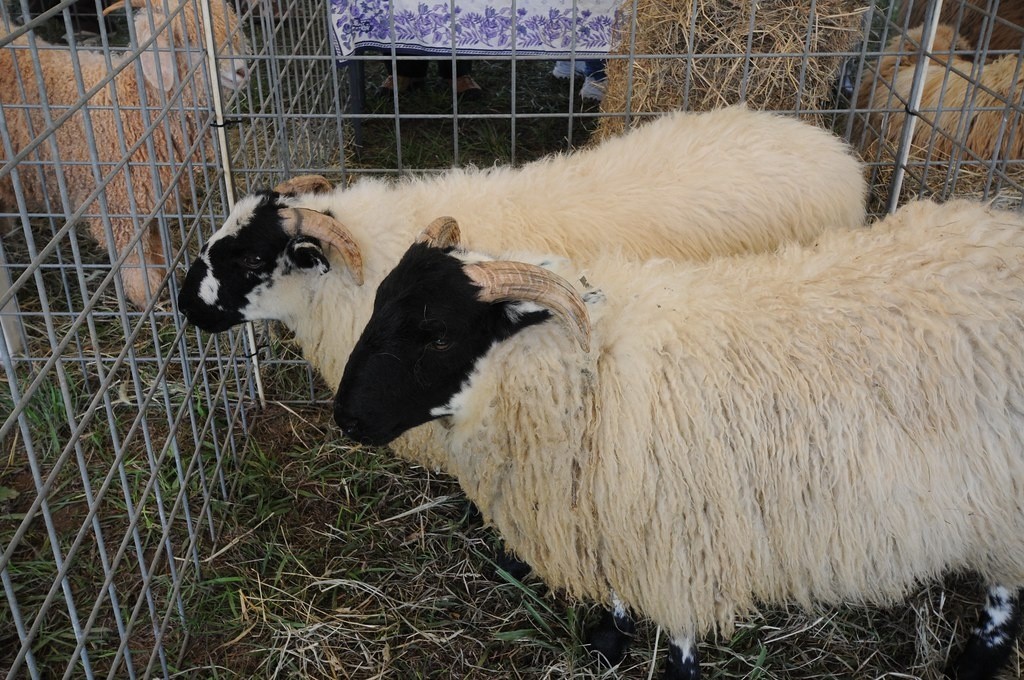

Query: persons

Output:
[550,59,609,101]
[380,47,484,102]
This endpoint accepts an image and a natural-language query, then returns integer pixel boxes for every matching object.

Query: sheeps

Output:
[0,0,256,314]
[327,196,1024,680]
[843,20,1024,163]
[177,100,871,478]
